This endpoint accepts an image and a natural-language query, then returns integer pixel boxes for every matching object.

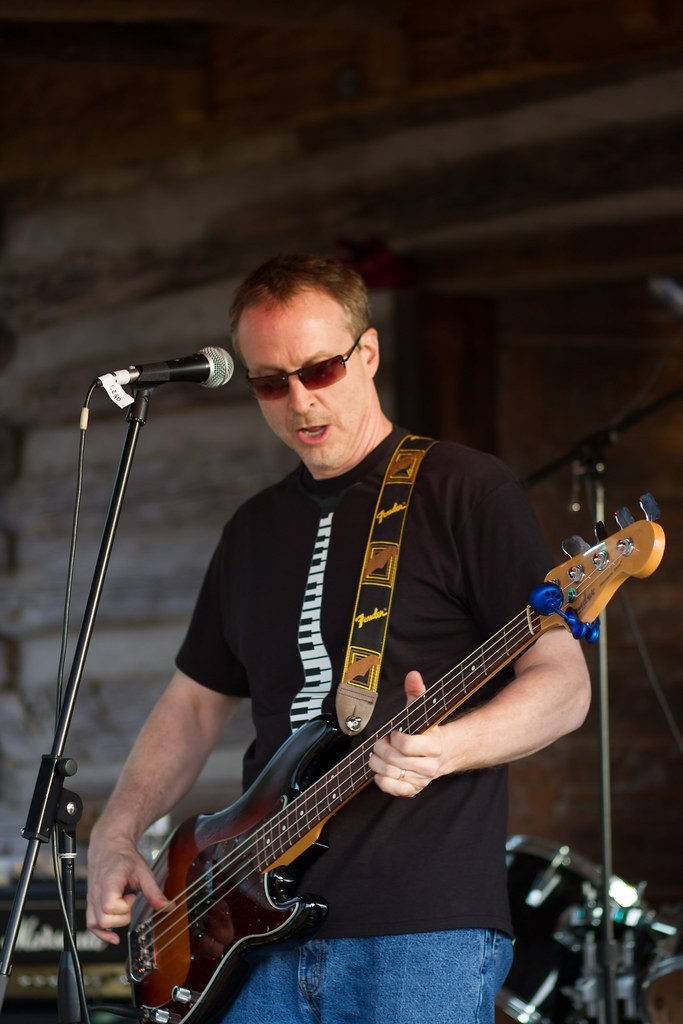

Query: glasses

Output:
[246,334,362,402]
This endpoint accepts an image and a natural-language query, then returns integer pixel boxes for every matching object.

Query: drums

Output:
[495,834,663,1024]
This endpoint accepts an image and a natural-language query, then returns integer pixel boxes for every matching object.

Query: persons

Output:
[86,252,589,1024]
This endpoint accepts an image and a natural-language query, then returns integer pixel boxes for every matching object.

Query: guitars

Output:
[125,493,666,1024]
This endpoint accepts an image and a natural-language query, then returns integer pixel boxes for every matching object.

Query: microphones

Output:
[651,277,683,313]
[98,344,235,389]
